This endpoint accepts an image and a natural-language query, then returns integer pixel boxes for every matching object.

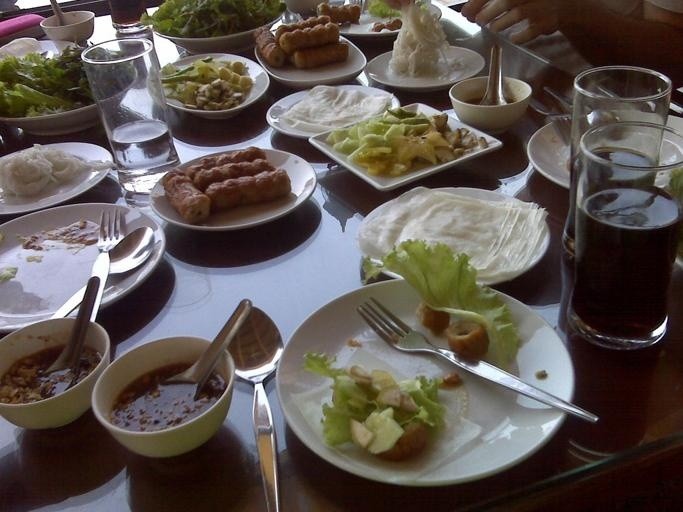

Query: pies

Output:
[358,186,551,282]
[278,85,389,132]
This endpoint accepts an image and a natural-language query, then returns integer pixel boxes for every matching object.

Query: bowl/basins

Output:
[447,77,532,135]
[0,318,113,430]
[90,335,235,461]
[37,10,95,40]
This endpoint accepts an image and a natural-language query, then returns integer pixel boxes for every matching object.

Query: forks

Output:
[357,298,601,425]
[81,208,127,330]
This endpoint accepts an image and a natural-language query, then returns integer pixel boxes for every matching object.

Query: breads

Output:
[256,1,403,72]
[161,145,292,223]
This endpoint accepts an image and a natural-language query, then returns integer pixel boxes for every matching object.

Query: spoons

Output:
[50,225,157,323]
[226,305,284,512]
[167,300,251,396]
[45,279,102,387]
[482,42,508,104]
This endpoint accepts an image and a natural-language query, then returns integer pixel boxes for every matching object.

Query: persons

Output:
[461,0,683,87]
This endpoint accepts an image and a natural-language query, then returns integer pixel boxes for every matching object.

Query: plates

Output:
[157,52,270,121]
[274,278,579,490]
[353,184,553,286]
[254,35,368,85]
[283,2,445,35]
[266,84,400,139]
[3,36,139,138]
[303,102,504,193]
[1,204,167,326]
[527,116,682,195]
[148,1,283,50]
[0,139,112,217]
[363,47,486,91]
[150,148,318,234]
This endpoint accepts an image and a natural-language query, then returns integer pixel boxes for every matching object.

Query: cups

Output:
[105,1,151,34]
[78,37,181,195]
[562,64,673,257]
[570,119,681,353]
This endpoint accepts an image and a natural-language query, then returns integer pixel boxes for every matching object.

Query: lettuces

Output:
[144,1,291,42]
[305,352,445,452]
[370,238,521,385]
[1,46,136,122]
